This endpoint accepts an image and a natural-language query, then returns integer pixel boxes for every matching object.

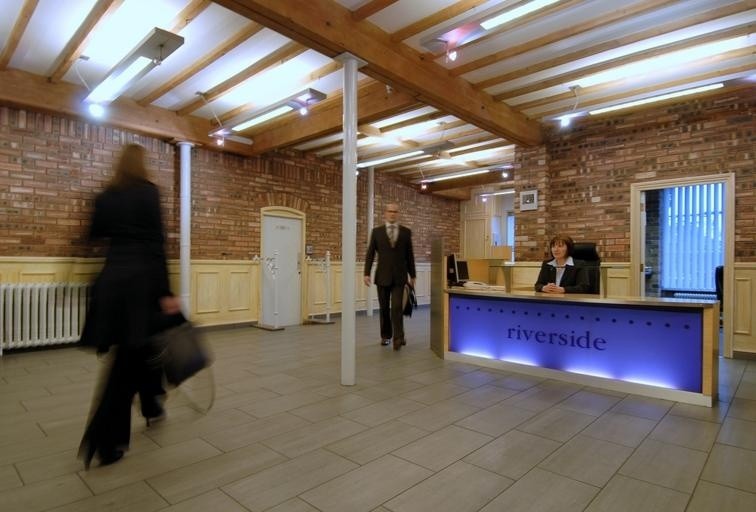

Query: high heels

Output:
[84,438,123,470]
[140,399,163,427]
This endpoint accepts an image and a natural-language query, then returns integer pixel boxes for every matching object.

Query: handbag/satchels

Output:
[403,283,417,317]
[146,321,205,386]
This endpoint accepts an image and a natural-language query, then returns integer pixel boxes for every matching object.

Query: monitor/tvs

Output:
[457,261,469,282]
[447,252,457,286]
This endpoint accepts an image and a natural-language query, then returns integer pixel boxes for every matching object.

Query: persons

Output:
[65,141,181,469]
[362,202,417,351]
[534,233,590,294]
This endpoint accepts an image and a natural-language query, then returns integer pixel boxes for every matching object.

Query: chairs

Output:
[548,240,602,295]
[713,265,727,334]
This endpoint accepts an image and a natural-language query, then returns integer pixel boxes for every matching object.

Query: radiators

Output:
[1,279,97,352]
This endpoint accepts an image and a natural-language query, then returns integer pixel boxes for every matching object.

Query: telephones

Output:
[463,281,487,289]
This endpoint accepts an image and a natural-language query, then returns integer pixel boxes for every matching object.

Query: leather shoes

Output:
[393,336,404,350]
[381,338,390,345]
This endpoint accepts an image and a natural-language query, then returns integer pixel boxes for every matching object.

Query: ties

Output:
[388,226,396,247]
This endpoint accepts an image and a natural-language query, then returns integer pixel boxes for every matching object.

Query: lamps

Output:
[78,25,185,117]
[418,0,566,64]
[195,88,327,147]
